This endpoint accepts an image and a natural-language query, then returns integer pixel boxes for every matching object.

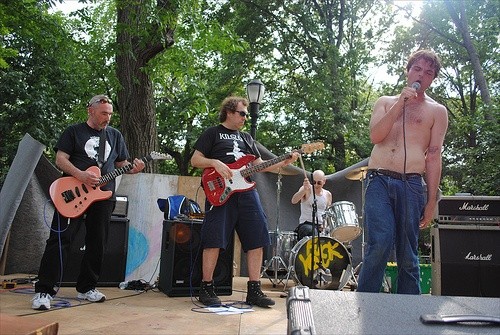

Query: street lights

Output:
[246,78,267,143]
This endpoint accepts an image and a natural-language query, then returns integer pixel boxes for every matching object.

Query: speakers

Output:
[57,217,129,287]
[431,196,500,298]
[158,220,234,297]
[285,286,500,335]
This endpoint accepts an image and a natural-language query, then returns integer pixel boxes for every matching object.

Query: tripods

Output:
[260,167,298,286]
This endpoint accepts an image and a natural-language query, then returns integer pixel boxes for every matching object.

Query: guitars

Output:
[49,150,172,219]
[201,142,326,206]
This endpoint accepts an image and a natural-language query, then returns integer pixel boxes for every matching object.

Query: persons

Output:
[355,49,449,295]
[291,170,332,243]
[190,96,299,310]
[30,95,144,310]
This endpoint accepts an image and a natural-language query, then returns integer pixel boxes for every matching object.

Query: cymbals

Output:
[345,166,370,181]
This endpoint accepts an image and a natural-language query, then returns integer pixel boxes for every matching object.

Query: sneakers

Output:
[76,288,106,303]
[32,292,52,309]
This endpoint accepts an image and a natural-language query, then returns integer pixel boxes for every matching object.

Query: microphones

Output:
[316,262,330,274]
[404,81,421,101]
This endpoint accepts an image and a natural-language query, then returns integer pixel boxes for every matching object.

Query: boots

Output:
[198,279,222,307]
[246,281,275,307]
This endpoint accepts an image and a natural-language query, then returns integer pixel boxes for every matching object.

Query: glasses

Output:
[88,98,113,107]
[229,108,250,119]
[312,180,325,185]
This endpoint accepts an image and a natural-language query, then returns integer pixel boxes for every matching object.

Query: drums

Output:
[264,230,297,273]
[289,234,353,291]
[326,201,362,241]
